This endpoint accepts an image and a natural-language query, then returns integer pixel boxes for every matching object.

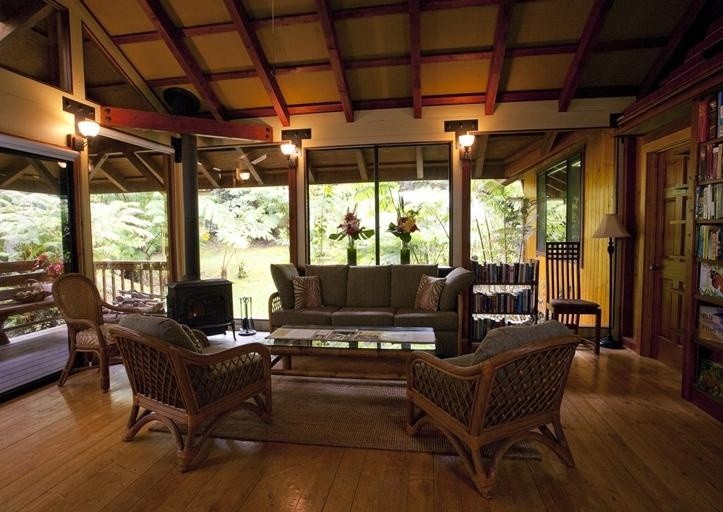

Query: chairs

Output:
[405,319,583,501]
[51,272,142,393]
[545,240,602,355]
[107,313,273,473]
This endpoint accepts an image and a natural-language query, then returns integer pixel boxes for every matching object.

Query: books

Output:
[692,91,723,400]
[469,259,535,343]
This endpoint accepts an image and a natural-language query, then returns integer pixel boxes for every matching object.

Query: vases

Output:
[347,248,357,266]
[401,249,410,265]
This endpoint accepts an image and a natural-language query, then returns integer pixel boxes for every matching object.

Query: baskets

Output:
[13,279,51,303]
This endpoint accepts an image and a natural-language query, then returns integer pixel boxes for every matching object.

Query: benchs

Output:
[0,259,65,343]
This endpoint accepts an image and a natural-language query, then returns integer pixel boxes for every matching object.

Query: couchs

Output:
[267,262,465,358]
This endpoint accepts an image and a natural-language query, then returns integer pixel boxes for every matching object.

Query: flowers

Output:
[386,195,423,249]
[328,202,375,248]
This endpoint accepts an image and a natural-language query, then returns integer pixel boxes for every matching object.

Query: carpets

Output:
[148,351,544,463]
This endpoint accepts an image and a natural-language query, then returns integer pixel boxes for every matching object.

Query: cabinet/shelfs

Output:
[680,87,723,421]
[469,259,540,353]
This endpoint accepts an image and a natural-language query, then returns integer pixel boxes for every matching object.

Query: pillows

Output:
[269,262,299,310]
[414,274,447,312]
[291,274,324,311]
[438,267,476,312]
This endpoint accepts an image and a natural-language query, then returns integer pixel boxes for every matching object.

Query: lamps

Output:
[458,134,476,161]
[592,212,633,350]
[66,120,101,152]
